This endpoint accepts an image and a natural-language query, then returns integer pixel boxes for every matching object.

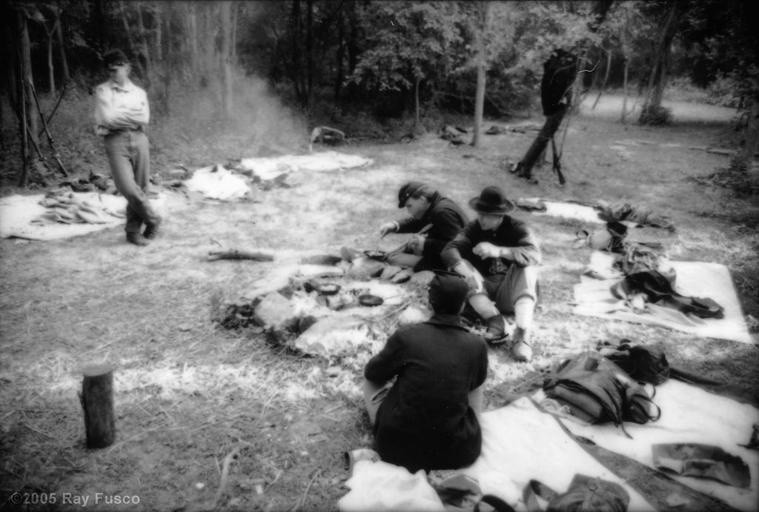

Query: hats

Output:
[103,49,127,64]
[429,272,469,316]
[398,182,426,209]
[469,186,516,216]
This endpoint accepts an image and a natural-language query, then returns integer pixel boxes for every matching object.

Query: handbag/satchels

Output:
[546,473,630,512]
[542,350,652,424]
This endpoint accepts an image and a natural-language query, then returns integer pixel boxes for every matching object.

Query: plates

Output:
[359,294,384,306]
[315,283,340,295]
[364,250,386,257]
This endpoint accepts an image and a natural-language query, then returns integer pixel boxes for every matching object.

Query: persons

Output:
[94,48,162,246]
[380,181,470,275]
[440,185,540,362]
[363,272,487,471]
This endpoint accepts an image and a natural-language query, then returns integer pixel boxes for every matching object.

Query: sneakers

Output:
[484,315,511,344]
[512,326,531,362]
[143,219,162,239]
[127,231,149,246]
